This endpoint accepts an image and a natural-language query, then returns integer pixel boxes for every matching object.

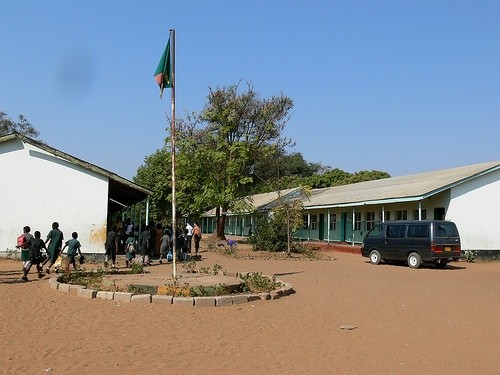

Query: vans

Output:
[360,220,461,269]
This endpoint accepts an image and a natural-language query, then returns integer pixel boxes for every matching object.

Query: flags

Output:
[154,33,173,99]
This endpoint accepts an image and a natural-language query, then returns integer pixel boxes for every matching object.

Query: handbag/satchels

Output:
[126,242,135,253]
[167,248,173,261]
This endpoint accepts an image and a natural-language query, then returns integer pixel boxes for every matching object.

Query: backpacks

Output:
[17,233,28,249]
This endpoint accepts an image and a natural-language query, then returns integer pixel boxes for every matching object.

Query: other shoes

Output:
[45,268,50,274]
[125,260,129,267]
[38,274,45,278]
[40,266,43,271]
[104,261,108,268]
[22,276,29,281]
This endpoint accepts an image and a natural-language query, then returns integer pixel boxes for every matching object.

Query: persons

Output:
[15,222,85,281]
[104,214,201,268]
